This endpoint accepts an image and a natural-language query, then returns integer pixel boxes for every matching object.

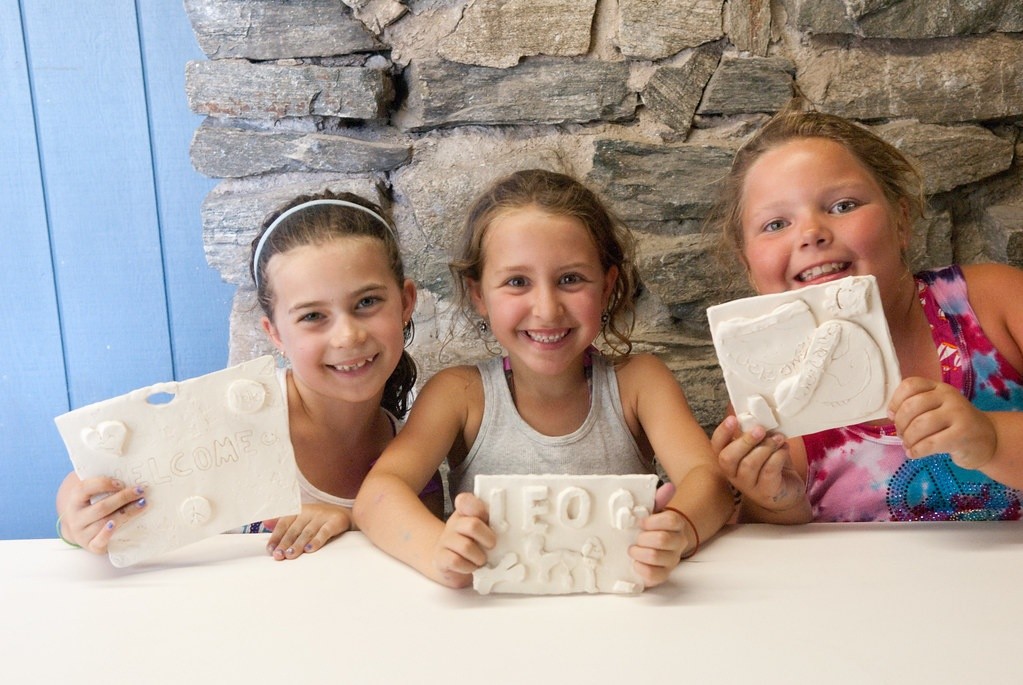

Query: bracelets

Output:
[56,514,81,548]
[664,506,700,560]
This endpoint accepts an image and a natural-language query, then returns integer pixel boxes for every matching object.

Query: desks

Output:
[3,517,1021,683]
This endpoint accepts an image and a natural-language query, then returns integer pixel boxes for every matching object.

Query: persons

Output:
[57,188,445,561]
[352,166,735,590]
[710,106,1023,525]
